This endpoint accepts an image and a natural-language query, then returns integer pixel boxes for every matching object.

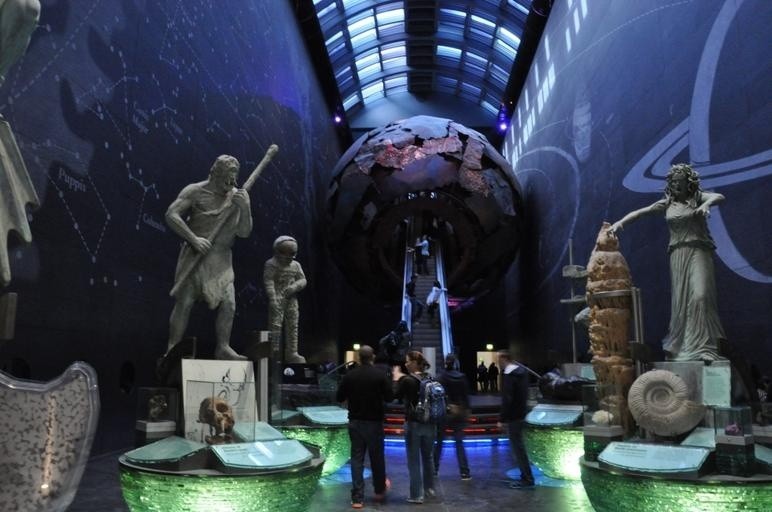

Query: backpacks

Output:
[409,373,447,425]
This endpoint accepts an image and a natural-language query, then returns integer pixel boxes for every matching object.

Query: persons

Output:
[406,273,425,327]
[498,353,535,489]
[380,321,415,405]
[264,234,308,364]
[407,237,422,275]
[335,345,401,509]
[163,154,255,362]
[432,353,475,482]
[394,350,436,505]
[477,360,499,393]
[414,234,430,276]
[426,281,449,328]
[606,163,729,362]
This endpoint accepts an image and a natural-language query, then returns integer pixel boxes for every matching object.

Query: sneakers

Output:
[426,488,435,497]
[461,473,472,481]
[433,471,439,478]
[374,479,391,499]
[407,496,424,504]
[352,501,363,508]
[511,478,536,489]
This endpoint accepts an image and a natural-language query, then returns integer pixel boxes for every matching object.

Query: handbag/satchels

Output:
[447,404,473,419]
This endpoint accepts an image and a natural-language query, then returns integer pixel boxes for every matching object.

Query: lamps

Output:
[497,95,514,134]
[332,99,344,126]
